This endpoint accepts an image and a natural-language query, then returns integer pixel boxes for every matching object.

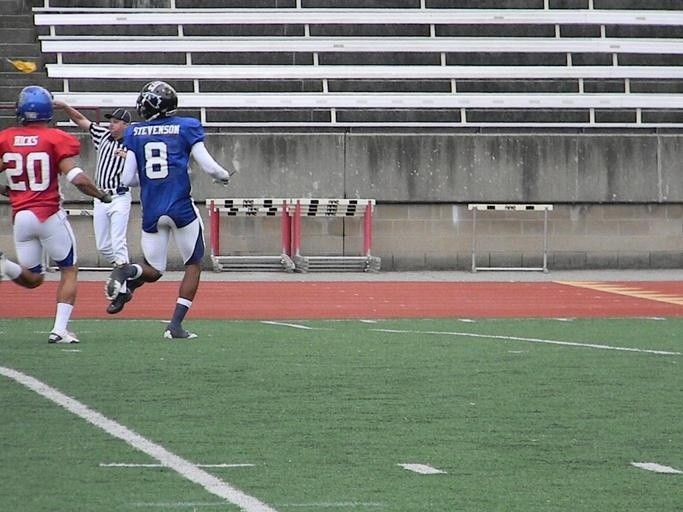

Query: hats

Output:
[102,106,132,125]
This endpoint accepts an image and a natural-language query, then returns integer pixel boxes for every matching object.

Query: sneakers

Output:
[47,329,81,346]
[105,260,145,316]
[0,249,8,281]
[162,322,199,341]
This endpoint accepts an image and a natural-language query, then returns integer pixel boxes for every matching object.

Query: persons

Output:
[104,81,230,339]
[52,100,138,314]
[0,85,112,343]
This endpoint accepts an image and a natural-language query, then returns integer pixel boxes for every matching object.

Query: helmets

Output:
[15,84,55,126]
[133,80,181,123]
